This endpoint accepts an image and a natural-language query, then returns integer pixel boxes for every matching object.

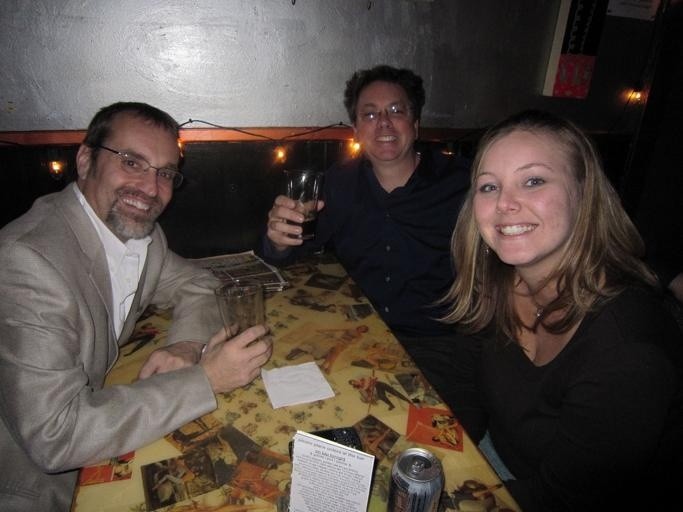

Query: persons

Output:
[418,110,681,512]
[265,66,497,448]
[1,103,274,511]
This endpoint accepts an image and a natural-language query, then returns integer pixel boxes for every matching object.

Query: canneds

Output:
[388,447,445,512]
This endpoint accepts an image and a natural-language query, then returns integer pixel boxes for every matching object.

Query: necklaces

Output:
[536,311,541,318]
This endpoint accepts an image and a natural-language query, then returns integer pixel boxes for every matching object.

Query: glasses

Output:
[98,145,183,190]
[359,102,416,122]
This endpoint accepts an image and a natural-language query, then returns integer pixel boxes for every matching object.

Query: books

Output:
[184,250,289,292]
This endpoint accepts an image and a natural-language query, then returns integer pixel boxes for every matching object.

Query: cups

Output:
[279,169,323,243]
[214,278,268,346]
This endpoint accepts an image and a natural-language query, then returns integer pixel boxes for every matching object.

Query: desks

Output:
[69,241,520,512]
